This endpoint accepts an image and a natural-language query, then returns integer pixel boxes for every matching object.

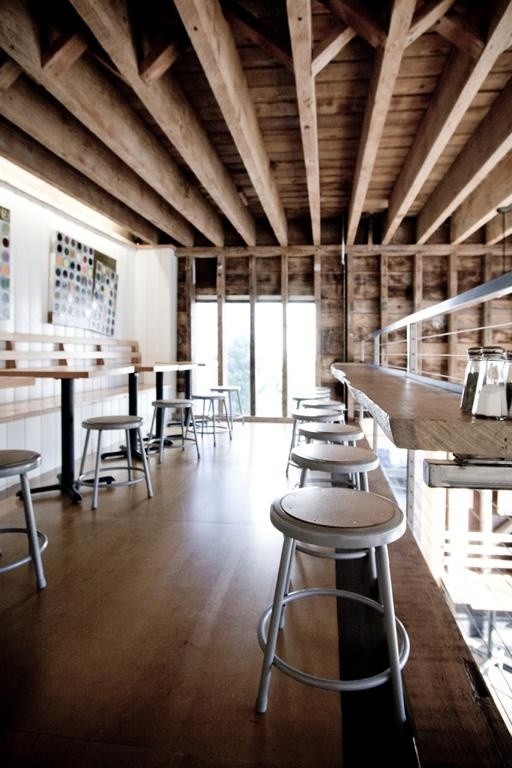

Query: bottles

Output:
[461,347,512,419]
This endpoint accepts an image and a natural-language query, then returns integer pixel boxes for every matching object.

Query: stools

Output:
[292,448,380,578]
[186,394,231,446]
[254,485,410,723]
[146,396,203,463]
[77,417,154,511]
[284,388,354,478]
[2,450,49,590]
[206,386,246,428]
[298,421,365,487]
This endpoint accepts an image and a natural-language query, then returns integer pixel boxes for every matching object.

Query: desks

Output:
[330,363,391,383]
[343,376,510,490]
[1,332,205,503]
